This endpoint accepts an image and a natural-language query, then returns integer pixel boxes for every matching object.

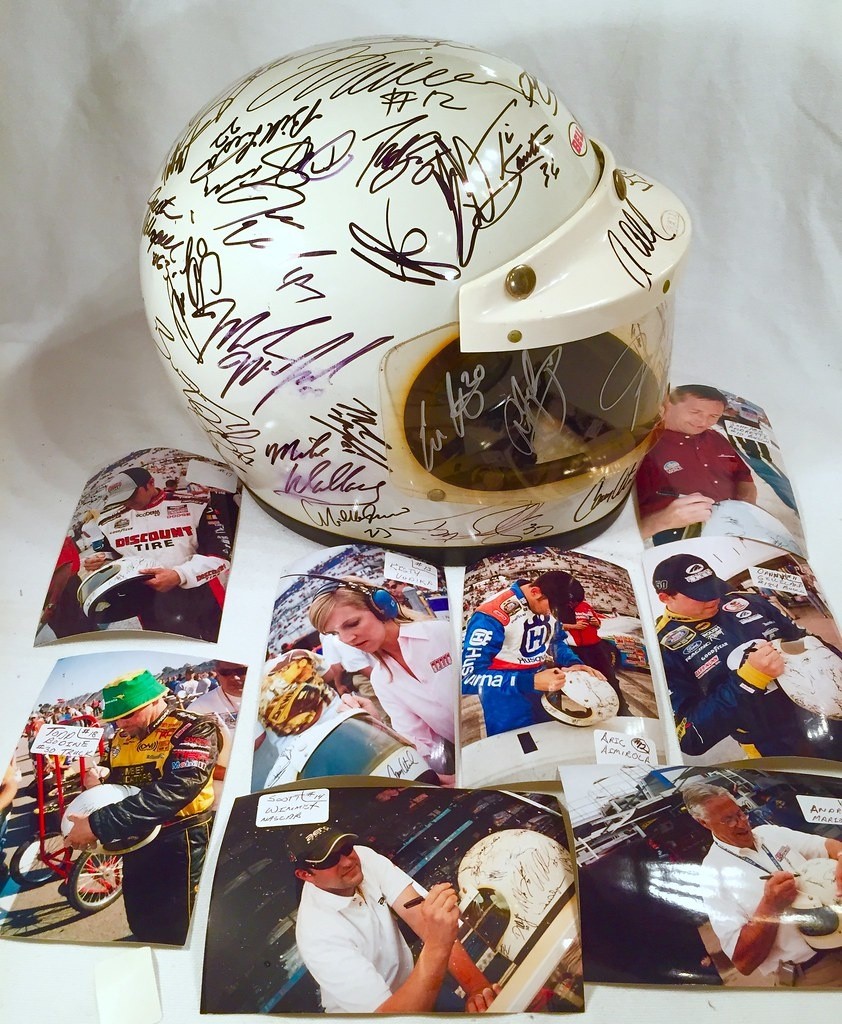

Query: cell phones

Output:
[517,731,538,753]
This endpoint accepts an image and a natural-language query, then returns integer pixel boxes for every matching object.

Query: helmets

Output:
[60,783,162,855]
[137,38,693,565]
[453,829,578,966]
[263,707,440,785]
[791,858,842,950]
[77,555,166,624]
[540,670,619,727]
[770,635,842,722]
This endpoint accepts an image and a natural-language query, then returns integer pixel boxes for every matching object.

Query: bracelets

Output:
[47,602,55,608]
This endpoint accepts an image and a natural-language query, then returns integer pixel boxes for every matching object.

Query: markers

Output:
[83,556,114,562]
[760,872,803,882]
[351,690,361,708]
[654,489,722,506]
[91,759,105,787]
[743,648,778,653]
[553,669,557,673]
[403,882,455,910]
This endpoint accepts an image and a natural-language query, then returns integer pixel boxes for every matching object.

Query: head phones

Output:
[311,581,401,622]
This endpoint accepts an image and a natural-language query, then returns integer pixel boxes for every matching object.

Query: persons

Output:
[35,467,239,643]
[460,548,641,654]
[285,822,503,1015]
[183,660,248,821]
[0,670,220,877]
[682,782,842,989]
[461,571,607,738]
[63,669,225,947]
[556,597,635,716]
[718,393,773,463]
[652,552,842,764]
[308,576,456,789]
[631,384,757,547]
[254,578,414,755]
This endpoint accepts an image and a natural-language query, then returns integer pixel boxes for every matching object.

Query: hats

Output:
[542,570,585,624]
[653,553,733,602]
[103,467,152,508]
[289,824,359,865]
[101,669,170,722]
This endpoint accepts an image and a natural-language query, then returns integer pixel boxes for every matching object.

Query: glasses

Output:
[121,705,150,719]
[304,841,354,871]
[217,667,247,676]
[705,807,748,828]
